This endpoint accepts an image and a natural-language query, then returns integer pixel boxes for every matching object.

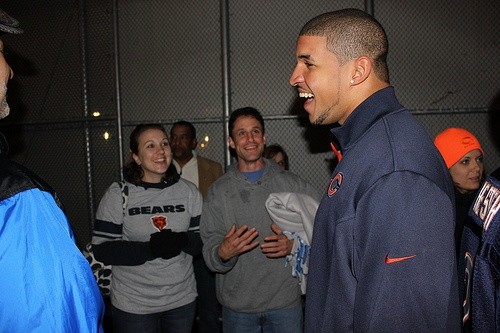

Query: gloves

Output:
[148,231,187,260]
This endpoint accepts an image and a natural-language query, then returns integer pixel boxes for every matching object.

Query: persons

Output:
[199,107,318,333]
[90,124,205,333]
[290,8,462,333]
[264,144,289,171]
[436,89,500,333]
[0,10,106,333]
[170,121,222,333]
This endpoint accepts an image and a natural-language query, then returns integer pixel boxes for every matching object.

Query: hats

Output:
[434,127,484,169]
